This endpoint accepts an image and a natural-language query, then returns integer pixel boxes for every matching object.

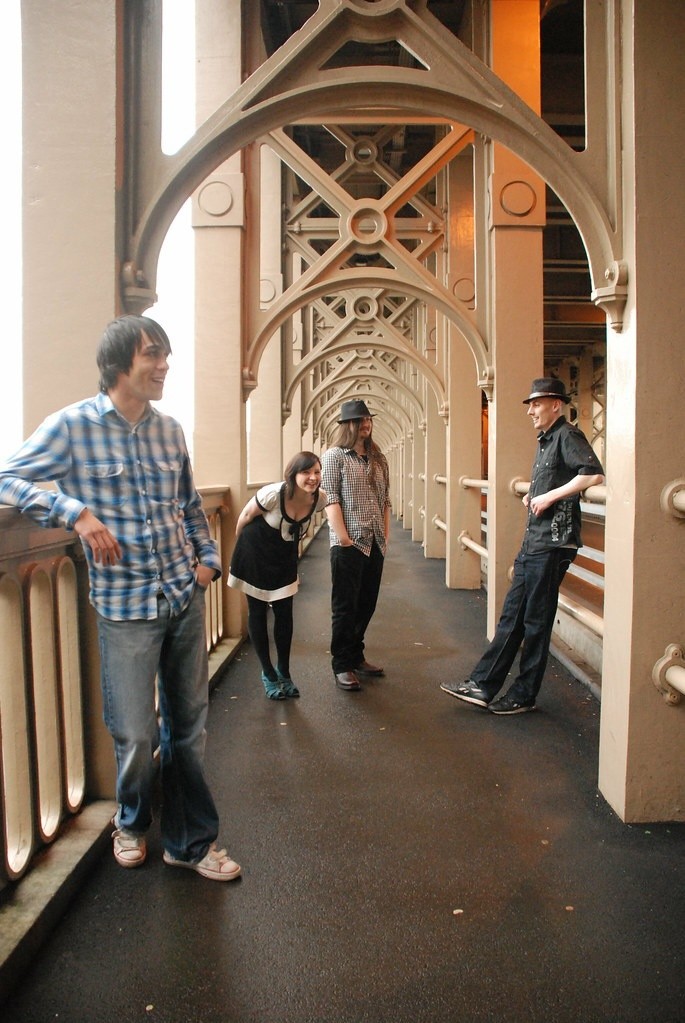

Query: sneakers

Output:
[439,681,491,708]
[112,814,148,868]
[165,846,243,883]
[486,695,536,715]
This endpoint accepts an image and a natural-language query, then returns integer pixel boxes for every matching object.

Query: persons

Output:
[0,316,241,884]
[227,452,329,697]
[433,378,606,718]
[317,399,393,690]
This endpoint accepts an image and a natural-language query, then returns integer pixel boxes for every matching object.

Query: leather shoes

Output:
[335,672,360,689]
[356,661,385,675]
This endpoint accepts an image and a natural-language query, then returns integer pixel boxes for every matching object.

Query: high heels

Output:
[275,666,301,697]
[261,670,287,701]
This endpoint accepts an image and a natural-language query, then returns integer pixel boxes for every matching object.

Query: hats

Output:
[337,400,376,423]
[523,378,572,405]
